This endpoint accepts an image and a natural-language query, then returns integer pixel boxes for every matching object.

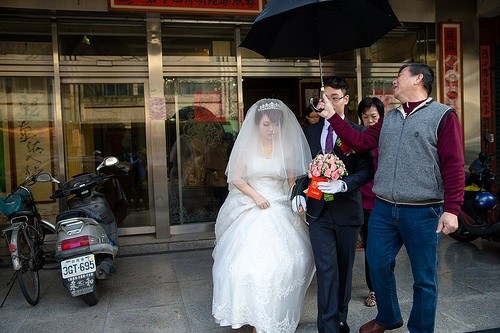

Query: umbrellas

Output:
[238,0,402,112]
[172,106,224,135]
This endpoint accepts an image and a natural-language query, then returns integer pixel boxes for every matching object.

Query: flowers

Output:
[308,153,348,202]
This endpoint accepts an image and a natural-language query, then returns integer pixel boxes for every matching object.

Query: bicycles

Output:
[0,168,58,309]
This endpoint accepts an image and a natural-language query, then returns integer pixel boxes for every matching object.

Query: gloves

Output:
[317,178,348,194]
[292,195,307,212]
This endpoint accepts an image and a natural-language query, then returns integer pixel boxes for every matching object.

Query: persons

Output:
[290,76,375,333]
[355,97,386,248]
[211,101,316,333]
[316,63,464,333]
[170,123,239,225]
[299,98,322,132]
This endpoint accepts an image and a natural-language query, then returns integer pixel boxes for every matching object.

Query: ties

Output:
[325,125,334,154]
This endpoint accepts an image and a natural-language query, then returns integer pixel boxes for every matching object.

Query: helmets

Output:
[475,191,498,210]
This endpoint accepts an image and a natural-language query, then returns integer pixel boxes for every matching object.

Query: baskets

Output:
[0,189,31,218]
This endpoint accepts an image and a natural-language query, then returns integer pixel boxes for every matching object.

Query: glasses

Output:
[327,96,344,102]
[309,116,319,118]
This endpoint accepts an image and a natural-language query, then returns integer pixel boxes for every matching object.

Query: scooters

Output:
[36,155,120,307]
[443,135,500,244]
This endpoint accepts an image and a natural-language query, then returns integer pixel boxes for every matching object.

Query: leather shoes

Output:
[359,317,403,333]
[339,321,350,333]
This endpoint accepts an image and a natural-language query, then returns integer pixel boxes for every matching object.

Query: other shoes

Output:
[365,291,377,307]
